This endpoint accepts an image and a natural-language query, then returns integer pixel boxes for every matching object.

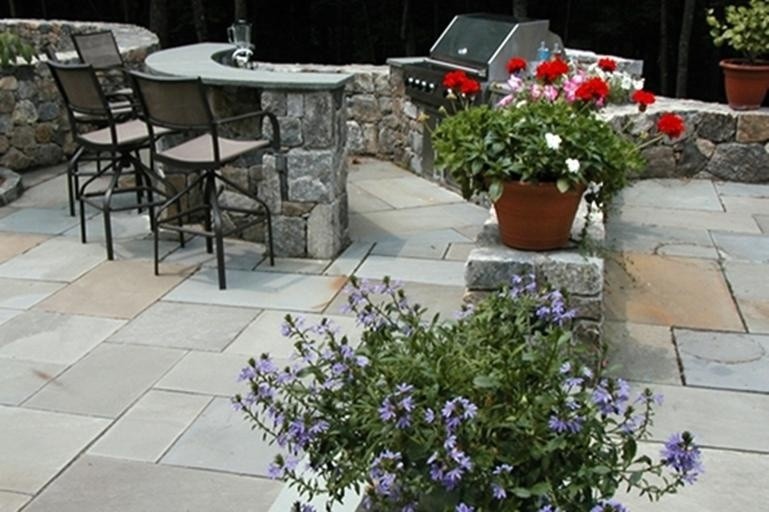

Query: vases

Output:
[492,180,580,253]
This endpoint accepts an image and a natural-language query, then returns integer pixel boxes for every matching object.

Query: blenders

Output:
[227,20,254,70]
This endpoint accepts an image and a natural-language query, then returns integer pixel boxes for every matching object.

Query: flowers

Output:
[415,54,691,210]
[215,261,710,512]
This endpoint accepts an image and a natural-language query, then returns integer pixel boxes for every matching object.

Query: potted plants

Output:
[704,0,768,112]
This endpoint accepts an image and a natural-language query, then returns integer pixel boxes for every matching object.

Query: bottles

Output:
[549,43,562,60]
[537,41,550,61]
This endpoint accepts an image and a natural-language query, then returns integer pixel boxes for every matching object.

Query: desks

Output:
[144,42,356,262]
